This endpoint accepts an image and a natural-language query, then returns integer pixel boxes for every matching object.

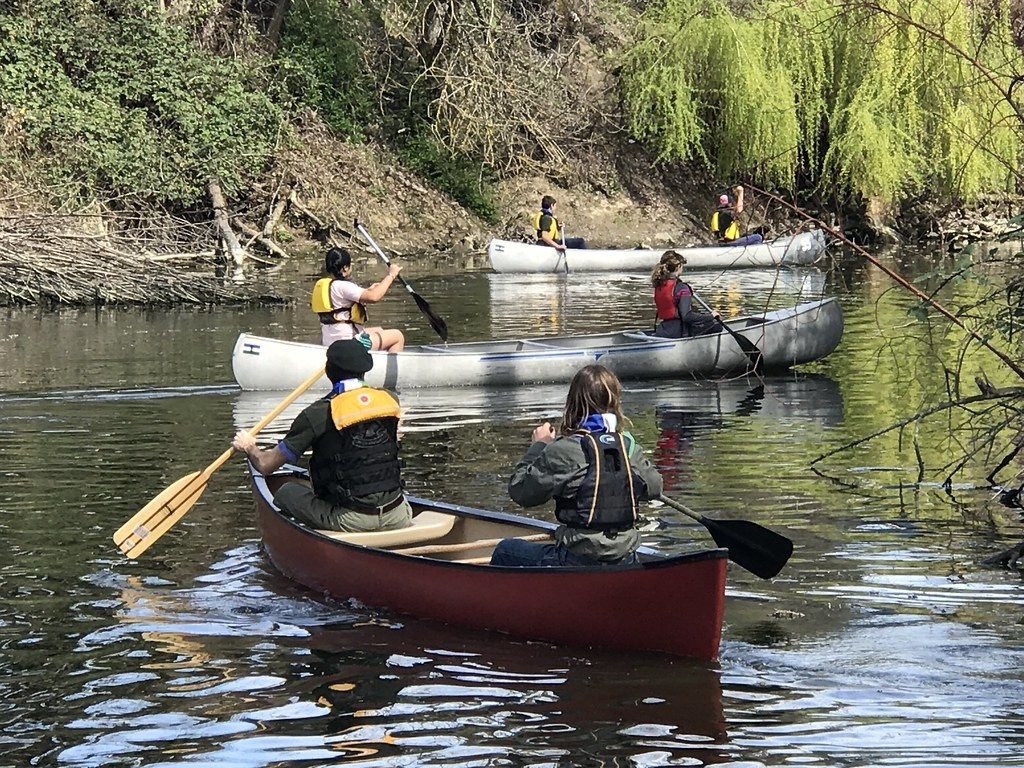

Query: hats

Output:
[715,194,734,208]
[327,339,373,372]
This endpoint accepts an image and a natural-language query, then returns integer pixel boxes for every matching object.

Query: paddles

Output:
[560,221,569,275]
[691,288,764,370]
[113,367,327,559]
[657,490,795,581]
[352,216,449,342]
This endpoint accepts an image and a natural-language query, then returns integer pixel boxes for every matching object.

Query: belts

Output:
[340,495,404,516]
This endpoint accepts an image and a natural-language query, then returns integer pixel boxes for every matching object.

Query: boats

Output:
[245,457,730,664]
[491,227,829,276]
[230,297,846,391]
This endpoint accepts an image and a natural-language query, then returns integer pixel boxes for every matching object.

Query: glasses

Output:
[676,261,686,266]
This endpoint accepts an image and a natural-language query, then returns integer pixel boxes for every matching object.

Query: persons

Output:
[536,194,586,249]
[230,339,413,532]
[711,186,770,246]
[311,248,404,352]
[490,366,664,567]
[651,252,723,338]
